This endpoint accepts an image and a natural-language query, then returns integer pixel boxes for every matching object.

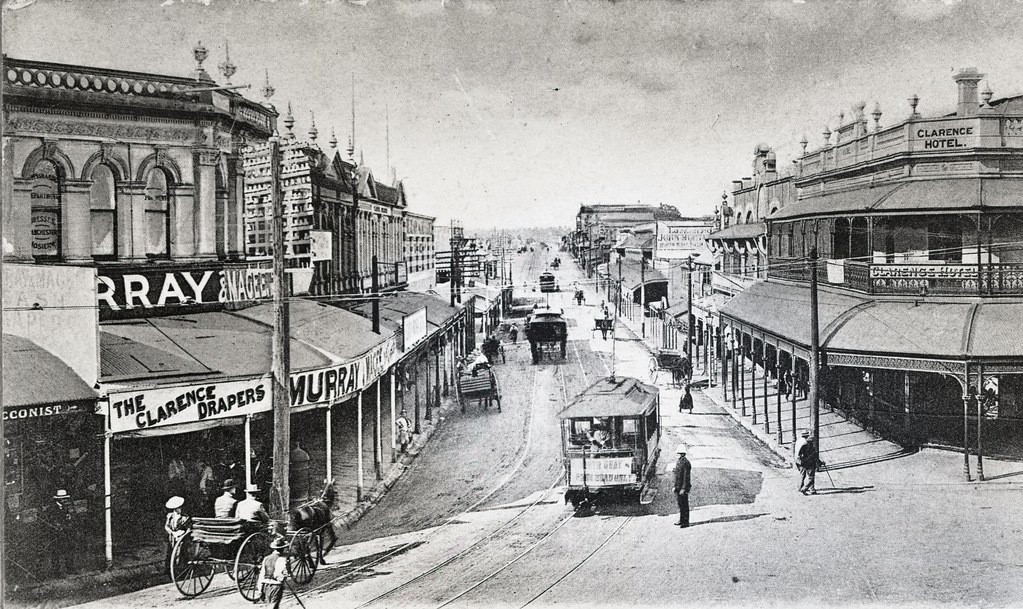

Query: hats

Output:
[269,536,290,548]
[53,490,70,499]
[221,479,237,489]
[244,484,261,492]
[801,429,811,436]
[399,410,407,415]
[165,496,184,509]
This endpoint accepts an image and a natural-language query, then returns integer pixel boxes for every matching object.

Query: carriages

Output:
[591,311,614,341]
[456,349,501,414]
[480,340,505,364]
[550,258,559,269]
[170,479,340,602]
[572,290,585,306]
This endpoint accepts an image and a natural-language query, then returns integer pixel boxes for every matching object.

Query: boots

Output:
[401,444,409,454]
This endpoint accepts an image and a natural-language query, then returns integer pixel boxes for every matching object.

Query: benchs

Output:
[192,517,260,552]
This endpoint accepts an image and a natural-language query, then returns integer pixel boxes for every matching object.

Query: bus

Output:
[527,306,567,356]
[539,271,555,292]
[556,376,660,514]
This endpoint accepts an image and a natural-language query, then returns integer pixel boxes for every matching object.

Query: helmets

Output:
[676,444,687,454]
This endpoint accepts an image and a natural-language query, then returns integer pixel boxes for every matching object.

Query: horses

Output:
[285,480,339,567]
[672,358,690,387]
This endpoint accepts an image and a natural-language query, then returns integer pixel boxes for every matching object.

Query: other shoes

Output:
[48,566,81,579]
[811,491,820,495]
[674,520,690,528]
[800,488,809,495]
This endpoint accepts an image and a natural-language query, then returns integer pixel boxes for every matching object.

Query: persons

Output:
[600,300,604,312]
[508,302,512,316]
[485,336,498,343]
[672,445,692,528]
[44,490,82,579]
[807,246,815,279]
[456,349,488,374]
[395,410,412,453]
[785,366,805,399]
[533,300,538,309]
[679,379,694,414]
[586,417,612,458]
[794,430,825,495]
[510,323,518,335]
[604,306,609,318]
[164,447,291,609]
